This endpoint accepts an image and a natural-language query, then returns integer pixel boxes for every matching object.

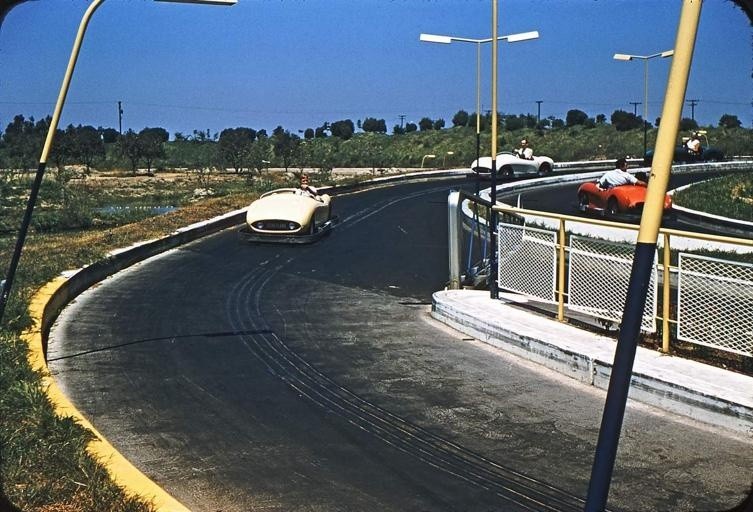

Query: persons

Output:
[293,172,318,200]
[511,136,534,162]
[594,158,648,189]
[682,131,702,152]
